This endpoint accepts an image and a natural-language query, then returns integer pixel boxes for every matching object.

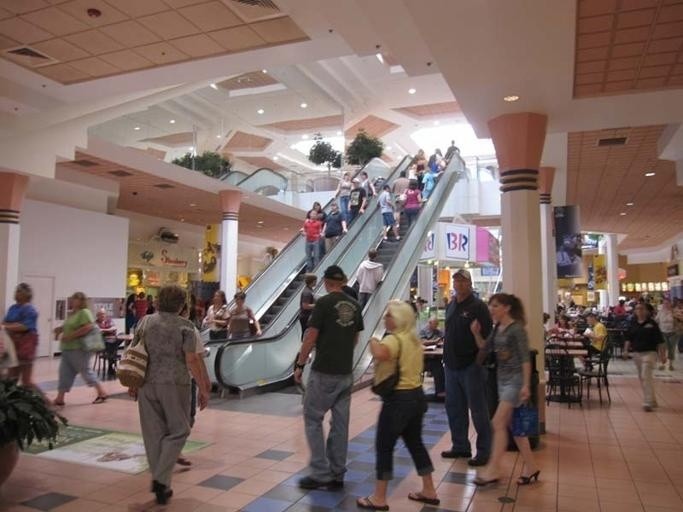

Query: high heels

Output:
[472,475,500,485]
[515,469,541,484]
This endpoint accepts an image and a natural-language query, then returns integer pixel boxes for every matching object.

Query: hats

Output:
[323,265,346,281]
[453,269,472,280]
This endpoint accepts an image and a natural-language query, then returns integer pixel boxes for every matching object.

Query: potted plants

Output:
[0,347,71,512]
[306,129,344,192]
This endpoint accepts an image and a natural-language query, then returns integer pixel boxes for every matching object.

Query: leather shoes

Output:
[331,474,345,488]
[441,449,472,457]
[176,451,192,468]
[155,487,174,504]
[299,475,336,490]
[146,479,167,491]
[468,453,488,465]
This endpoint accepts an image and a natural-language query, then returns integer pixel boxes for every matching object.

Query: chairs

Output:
[607,327,624,358]
[577,332,615,404]
[546,335,566,345]
[543,345,582,409]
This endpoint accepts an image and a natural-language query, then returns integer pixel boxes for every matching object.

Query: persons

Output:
[441,269,493,466]
[355,247,384,309]
[313,202,327,225]
[51,291,110,406]
[333,172,353,226]
[419,315,444,401]
[401,179,428,227]
[390,170,409,230]
[582,313,608,380]
[128,285,209,504]
[126,294,136,334]
[298,274,317,343]
[201,289,229,340]
[470,292,540,486]
[348,178,367,219]
[152,296,158,313]
[360,171,378,200]
[672,299,682,354]
[355,298,440,510]
[92,307,116,375]
[147,295,154,315]
[407,148,447,198]
[541,313,552,346]
[298,211,323,274]
[444,140,460,159]
[621,292,681,314]
[613,300,628,317]
[318,202,349,253]
[228,292,262,338]
[132,292,149,323]
[295,264,364,490]
[654,298,678,371]
[621,302,669,411]
[554,298,587,339]
[376,185,402,242]
[1,283,52,404]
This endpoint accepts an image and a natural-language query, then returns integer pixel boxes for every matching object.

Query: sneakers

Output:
[651,401,660,408]
[668,364,675,372]
[383,237,392,242]
[394,236,404,242]
[658,362,667,371]
[643,403,654,413]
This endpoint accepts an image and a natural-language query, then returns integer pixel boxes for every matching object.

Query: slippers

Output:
[91,392,108,405]
[407,491,441,504]
[52,398,66,406]
[357,496,389,511]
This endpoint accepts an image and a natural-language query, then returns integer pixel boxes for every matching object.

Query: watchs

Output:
[295,363,305,369]
